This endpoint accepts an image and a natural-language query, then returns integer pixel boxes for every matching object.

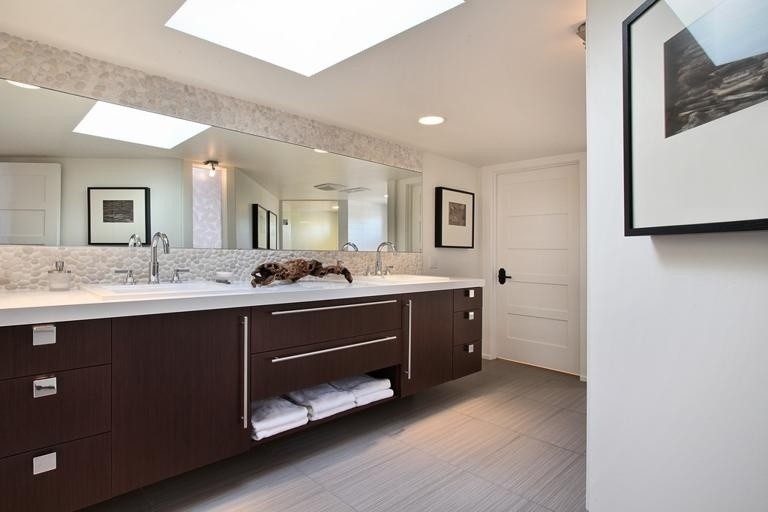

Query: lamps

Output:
[204,159,221,178]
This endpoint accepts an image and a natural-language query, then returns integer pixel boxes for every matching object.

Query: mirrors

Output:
[0,78,427,255]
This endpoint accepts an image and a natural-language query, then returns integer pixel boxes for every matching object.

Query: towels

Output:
[247,368,395,446]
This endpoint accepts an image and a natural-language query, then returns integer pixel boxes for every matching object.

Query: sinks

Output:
[352,274,451,284]
[78,283,251,297]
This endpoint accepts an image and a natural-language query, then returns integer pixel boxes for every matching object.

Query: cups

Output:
[216,271,233,286]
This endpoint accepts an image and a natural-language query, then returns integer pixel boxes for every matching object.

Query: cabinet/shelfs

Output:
[112,293,251,501]
[402,289,453,401]
[251,290,402,402]
[454,285,484,382]
[0,302,112,512]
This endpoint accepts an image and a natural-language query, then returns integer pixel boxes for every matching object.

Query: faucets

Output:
[148,231,170,284]
[128,233,142,248]
[374,241,397,276]
[342,242,358,251]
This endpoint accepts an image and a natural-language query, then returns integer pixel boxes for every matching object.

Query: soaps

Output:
[215,271,234,282]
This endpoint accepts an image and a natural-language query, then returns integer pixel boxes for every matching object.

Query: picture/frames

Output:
[267,209,278,249]
[252,203,268,248]
[85,185,153,245]
[618,1,768,239]
[433,185,477,251]
